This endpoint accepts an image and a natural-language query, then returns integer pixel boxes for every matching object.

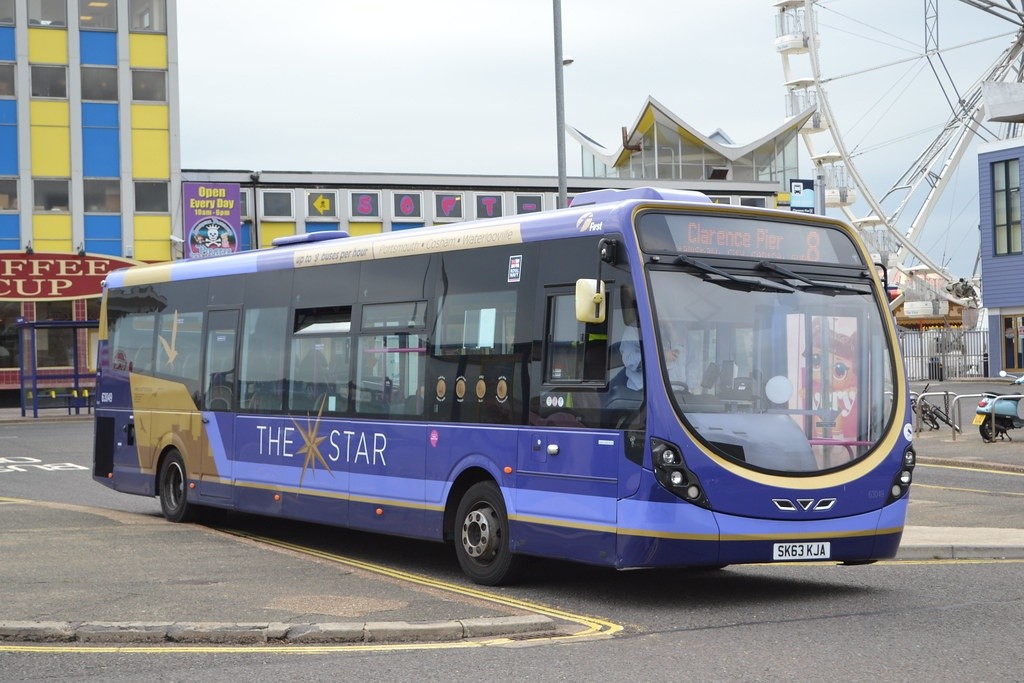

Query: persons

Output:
[619,298,687,390]
[222,337,422,416]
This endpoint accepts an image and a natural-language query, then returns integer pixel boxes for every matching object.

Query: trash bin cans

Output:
[929,358,939,379]
[983,353,987,377]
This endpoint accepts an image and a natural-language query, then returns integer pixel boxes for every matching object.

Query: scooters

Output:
[974,370,1023,442]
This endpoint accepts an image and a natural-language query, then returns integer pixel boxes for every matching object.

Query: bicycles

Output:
[909,381,959,432]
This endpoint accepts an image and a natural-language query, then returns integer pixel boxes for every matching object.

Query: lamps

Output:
[26,240,33,255]
[77,242,85,257]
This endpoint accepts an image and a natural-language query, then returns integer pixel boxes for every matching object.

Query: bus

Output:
[90,186,913,587]
